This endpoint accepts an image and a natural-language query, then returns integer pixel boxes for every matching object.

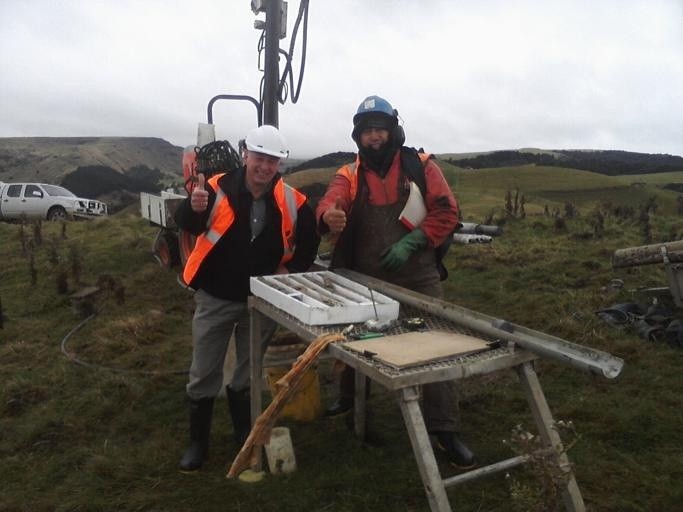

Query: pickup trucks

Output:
[0,181,110,225]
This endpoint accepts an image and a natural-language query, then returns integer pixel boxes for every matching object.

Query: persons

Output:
[312,93,477,473]
[171,125,322,473]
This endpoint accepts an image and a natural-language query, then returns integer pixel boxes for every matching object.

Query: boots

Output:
[225,386,251,456]
[180,397,215,474]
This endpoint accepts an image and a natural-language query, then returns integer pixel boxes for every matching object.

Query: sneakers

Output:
[437,432,476,470]
[325,397,355,419]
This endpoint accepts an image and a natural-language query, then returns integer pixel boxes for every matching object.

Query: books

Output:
[398,182,428,232]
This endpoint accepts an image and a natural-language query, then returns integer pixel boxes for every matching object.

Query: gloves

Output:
[377,229,428,273]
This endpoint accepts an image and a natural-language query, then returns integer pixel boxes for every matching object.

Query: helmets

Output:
[244,125,289,158]
[353,96,397,122]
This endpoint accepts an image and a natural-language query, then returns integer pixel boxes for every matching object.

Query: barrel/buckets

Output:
[319,350,356,419]
[261,331,323,423]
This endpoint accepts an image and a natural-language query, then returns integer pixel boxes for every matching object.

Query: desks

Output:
[247,294,587,512]
